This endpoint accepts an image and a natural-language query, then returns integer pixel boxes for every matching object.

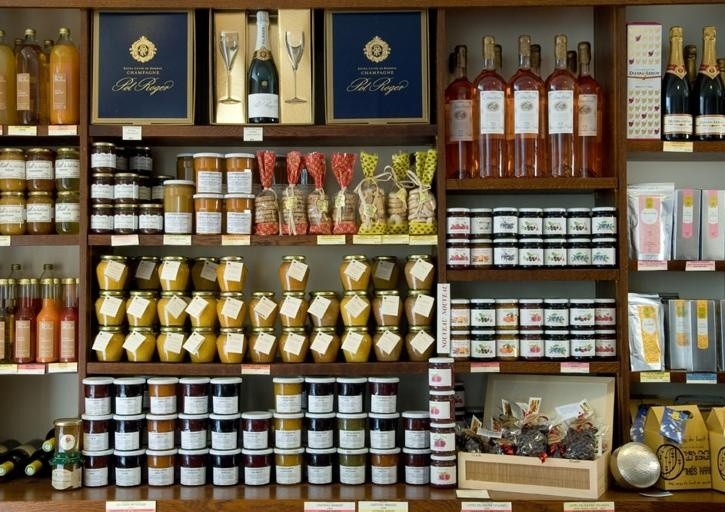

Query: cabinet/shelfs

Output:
[0,0,725,512]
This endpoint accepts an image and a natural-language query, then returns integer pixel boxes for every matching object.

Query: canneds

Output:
[0,146,78,237]
[49,358,459,491]
[448,299,616,361]
[89,140,254,235]
[91,255,435,362]
[445,206,618,269]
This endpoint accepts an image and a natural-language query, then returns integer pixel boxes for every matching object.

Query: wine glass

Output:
[283,28,309,104]
[214,28,243,107]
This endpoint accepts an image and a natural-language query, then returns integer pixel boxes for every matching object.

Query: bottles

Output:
[246,13,281,125]
[664,21,723,142]
[0,24,78,125]
[443,32,605,177]
[0,260,78,366]
[0,428,54,491]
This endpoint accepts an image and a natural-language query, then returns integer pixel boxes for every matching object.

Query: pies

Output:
[253,187,437,236]
